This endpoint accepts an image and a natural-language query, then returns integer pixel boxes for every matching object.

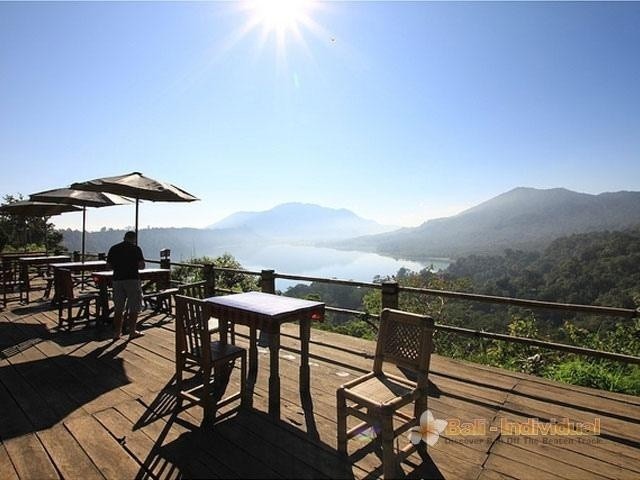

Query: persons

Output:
[106,231,145,341]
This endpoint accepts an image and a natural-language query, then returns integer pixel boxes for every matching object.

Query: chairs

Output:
[172,278,251,434]
[336,307,433,479]
[1,254,107,329]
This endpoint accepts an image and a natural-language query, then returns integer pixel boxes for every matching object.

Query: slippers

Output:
[113,329,121,340]
[129,331,145,338]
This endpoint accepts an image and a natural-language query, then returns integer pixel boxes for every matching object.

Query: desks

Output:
[203,291,327,395]
[91,267,172,325]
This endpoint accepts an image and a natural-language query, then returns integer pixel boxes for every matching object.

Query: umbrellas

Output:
[0,198,81,270]
[70,172,201,270]
[28,187,144,291]
